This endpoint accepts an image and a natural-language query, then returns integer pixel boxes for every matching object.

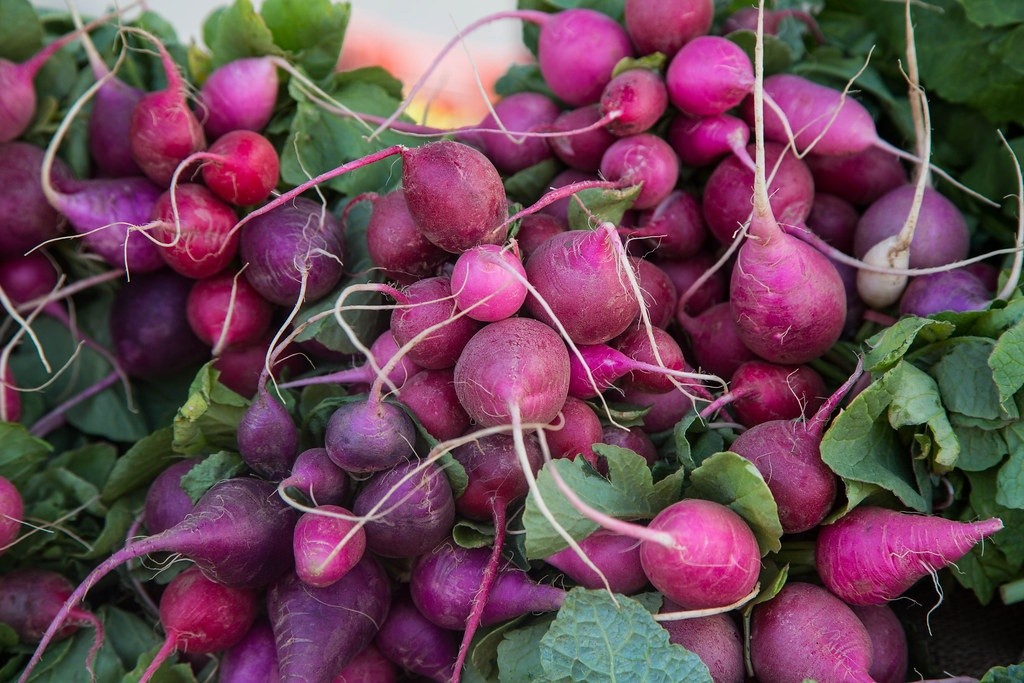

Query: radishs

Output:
[856,58,931,308]
